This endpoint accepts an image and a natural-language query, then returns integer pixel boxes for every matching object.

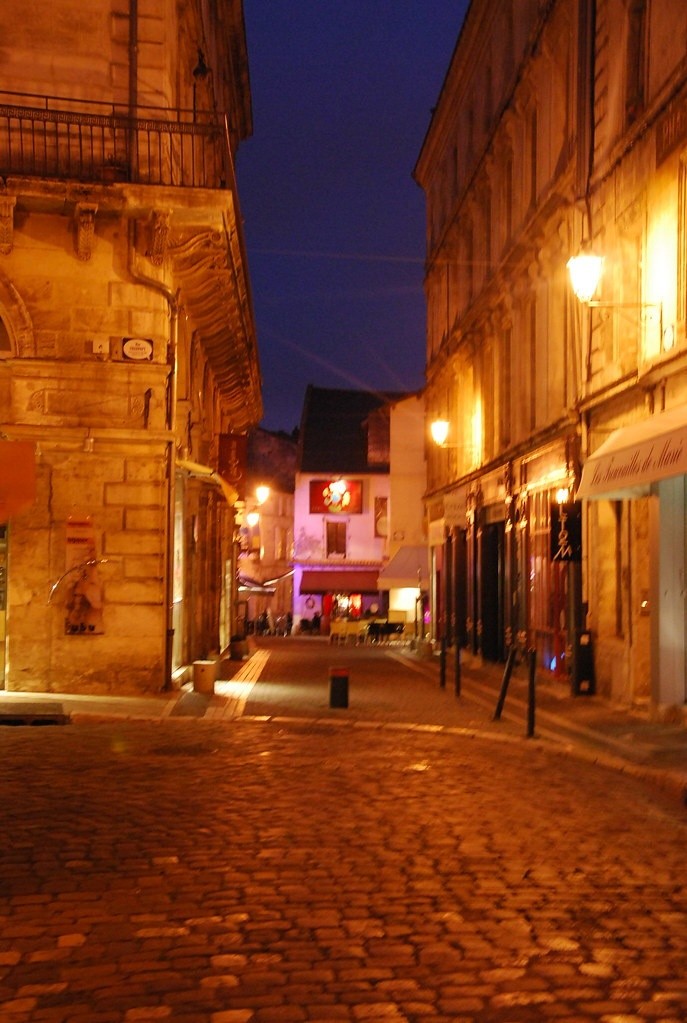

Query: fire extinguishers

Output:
[45,558,106,635]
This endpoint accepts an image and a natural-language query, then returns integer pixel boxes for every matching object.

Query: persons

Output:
[310,611,323,635]
[283,611,292,636]
[260,611,270,637]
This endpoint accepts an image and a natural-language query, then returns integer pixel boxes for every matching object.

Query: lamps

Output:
[430,412,462,449]
[564,238,663,353]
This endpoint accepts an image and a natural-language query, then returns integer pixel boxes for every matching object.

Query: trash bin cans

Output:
[230,634,243,660]
[192,661,217,696]
[328,665,351,709]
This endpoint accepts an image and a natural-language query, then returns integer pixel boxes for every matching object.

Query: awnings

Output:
[299,570,381,592]
[575,404,686,502]
[377,543,429,591]
[181,459,239,506]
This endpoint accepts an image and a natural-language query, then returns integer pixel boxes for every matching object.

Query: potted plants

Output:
[99,152,127,181]
[228,633,248,660]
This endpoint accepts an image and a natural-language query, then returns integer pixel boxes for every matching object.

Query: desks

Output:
[329,622,368,645]
[368,622,405,644]
